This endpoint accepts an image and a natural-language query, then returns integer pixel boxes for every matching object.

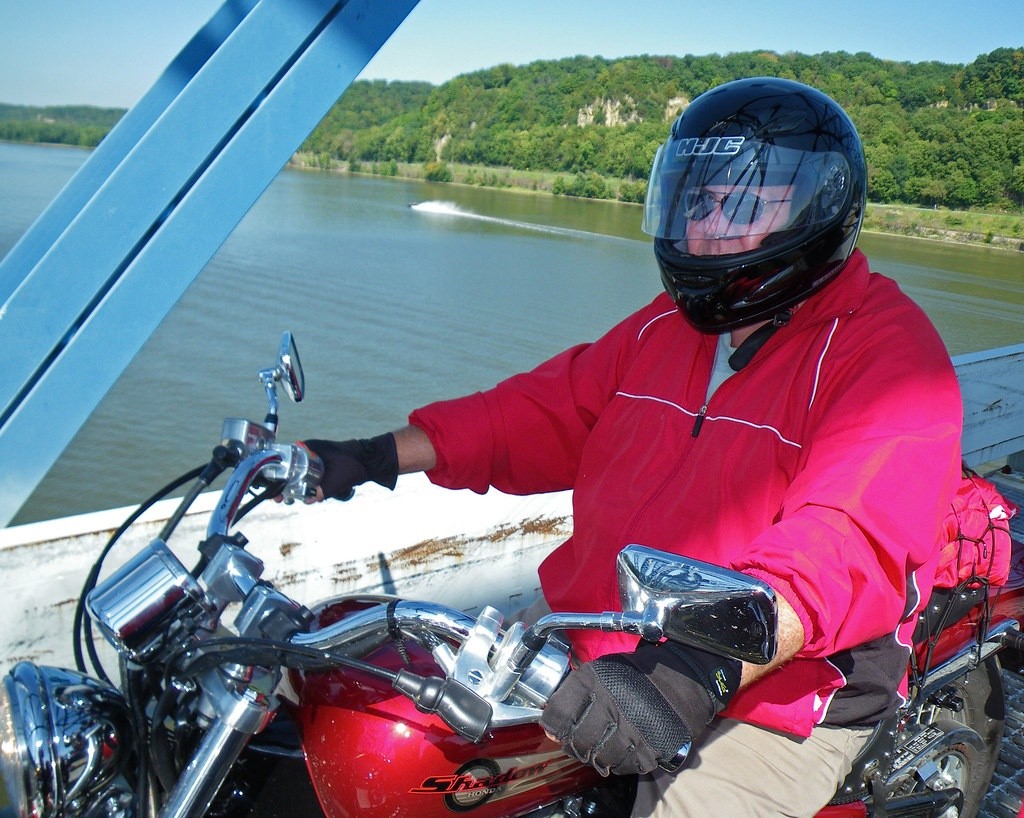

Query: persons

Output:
[275,76,963,818]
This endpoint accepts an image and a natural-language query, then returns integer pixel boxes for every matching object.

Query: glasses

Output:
[681,184,794,223]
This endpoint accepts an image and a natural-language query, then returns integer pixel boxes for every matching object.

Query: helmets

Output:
[642,76,866,333]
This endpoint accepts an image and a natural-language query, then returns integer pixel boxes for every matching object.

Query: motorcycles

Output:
[0,331,1024,817]
